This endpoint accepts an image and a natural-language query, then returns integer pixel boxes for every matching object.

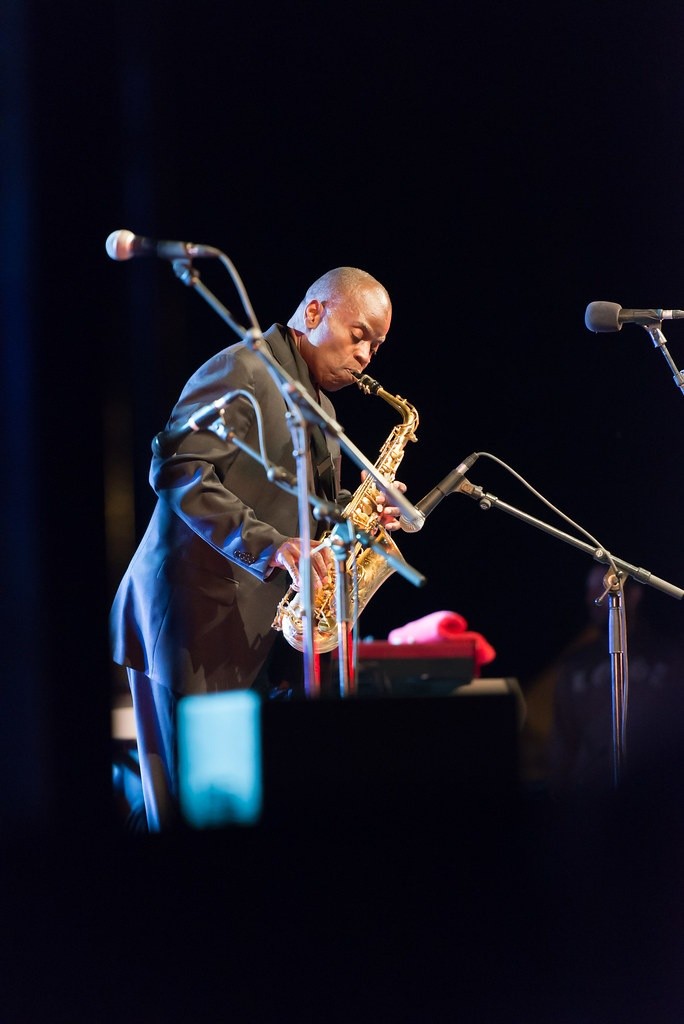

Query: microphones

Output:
[397,451,478,533]
[151,388,243,460]
[584,301,684,333]
[105,229,221,262]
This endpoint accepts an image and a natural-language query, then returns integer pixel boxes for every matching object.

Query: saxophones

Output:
[270,372,418,654]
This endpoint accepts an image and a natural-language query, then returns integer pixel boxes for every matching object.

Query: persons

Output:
[106,267,415,827]
